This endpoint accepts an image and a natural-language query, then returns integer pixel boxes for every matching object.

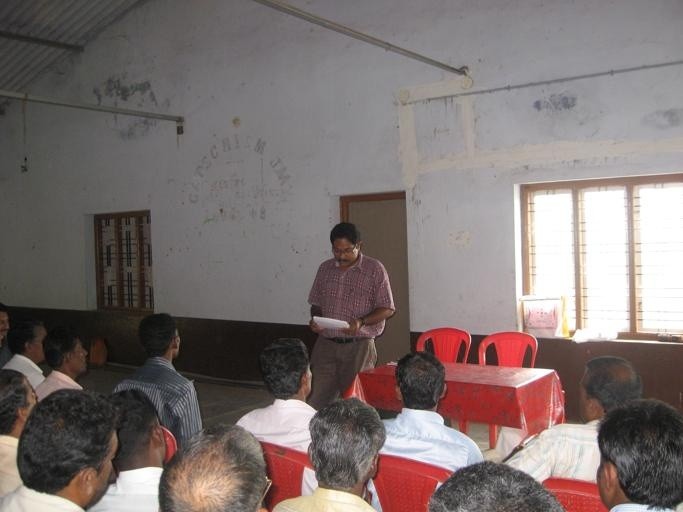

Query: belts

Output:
[319,333,376,346]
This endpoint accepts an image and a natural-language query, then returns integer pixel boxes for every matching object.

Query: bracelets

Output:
[360,316,365,327]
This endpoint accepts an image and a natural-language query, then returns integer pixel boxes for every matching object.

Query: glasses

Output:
[332,242,358,254]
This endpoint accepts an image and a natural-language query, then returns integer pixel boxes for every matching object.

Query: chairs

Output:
[258,441,314,512]
[374,453,453,512]
[459,331,538,450]
[160,425,177,462]
[415,328,471,363]
[539,478,610,512]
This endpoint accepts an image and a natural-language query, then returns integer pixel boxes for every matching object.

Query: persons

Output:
[234,337,319,497]
[1,300,202,511]
[595,397,683,512]
[504,355,644,487]
[426,459,567,512]
[305,221,397,411]
[270,395,387,512]
[364,350,485,512]
[157,421,269,512]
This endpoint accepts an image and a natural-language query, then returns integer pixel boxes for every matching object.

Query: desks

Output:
[358,363,566,449]
